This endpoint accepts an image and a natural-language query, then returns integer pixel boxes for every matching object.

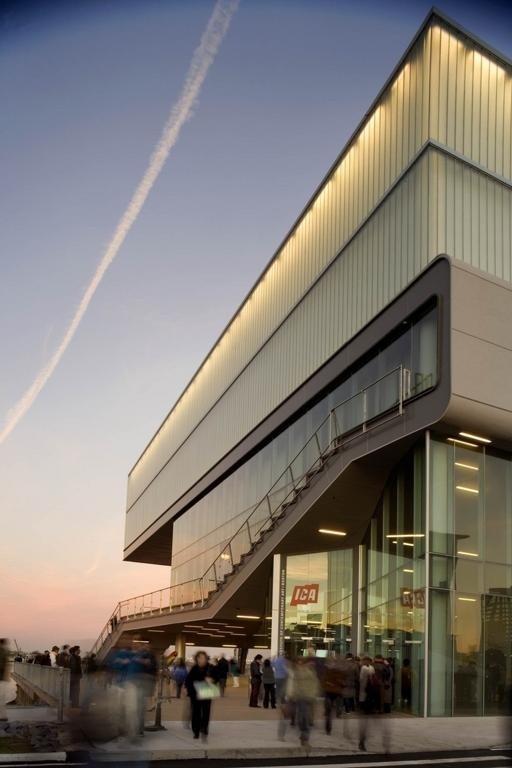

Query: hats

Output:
[360,654,374,663]
[344,652,353,658]
[52,645,60,651]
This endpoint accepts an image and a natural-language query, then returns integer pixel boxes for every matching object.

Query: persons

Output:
[401,658,413,711]
[454,661,506,709]
[1,634,400,755]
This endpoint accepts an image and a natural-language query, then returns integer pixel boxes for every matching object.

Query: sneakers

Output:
[249,703,261,708]
[263,703,277,709]
[192,732,208,742]
